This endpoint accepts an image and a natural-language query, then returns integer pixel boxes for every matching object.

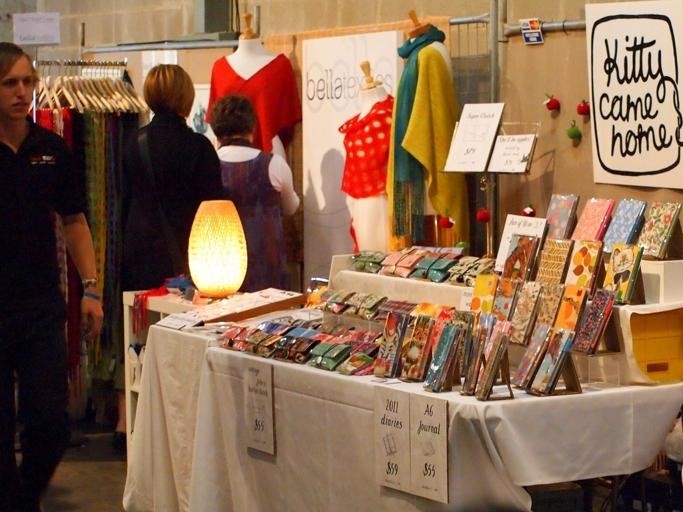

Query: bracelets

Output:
[81,291,102,300]
[81,276,100,289]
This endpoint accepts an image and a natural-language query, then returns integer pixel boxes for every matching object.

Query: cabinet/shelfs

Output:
[122,289,246,467]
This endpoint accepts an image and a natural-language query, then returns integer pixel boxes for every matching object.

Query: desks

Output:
[149,307,683,509]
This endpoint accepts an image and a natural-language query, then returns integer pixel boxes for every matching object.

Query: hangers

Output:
[28,58,149,116]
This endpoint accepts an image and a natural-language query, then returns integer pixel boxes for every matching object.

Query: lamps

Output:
[185,200,249,298]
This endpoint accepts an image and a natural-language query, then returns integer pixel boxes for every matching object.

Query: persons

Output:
[0,39,104,510]
[109,61,227,454]
[385,10,471,253]
[203,11,301,171]
[208,94,302,288]
[335,61,398,254]
